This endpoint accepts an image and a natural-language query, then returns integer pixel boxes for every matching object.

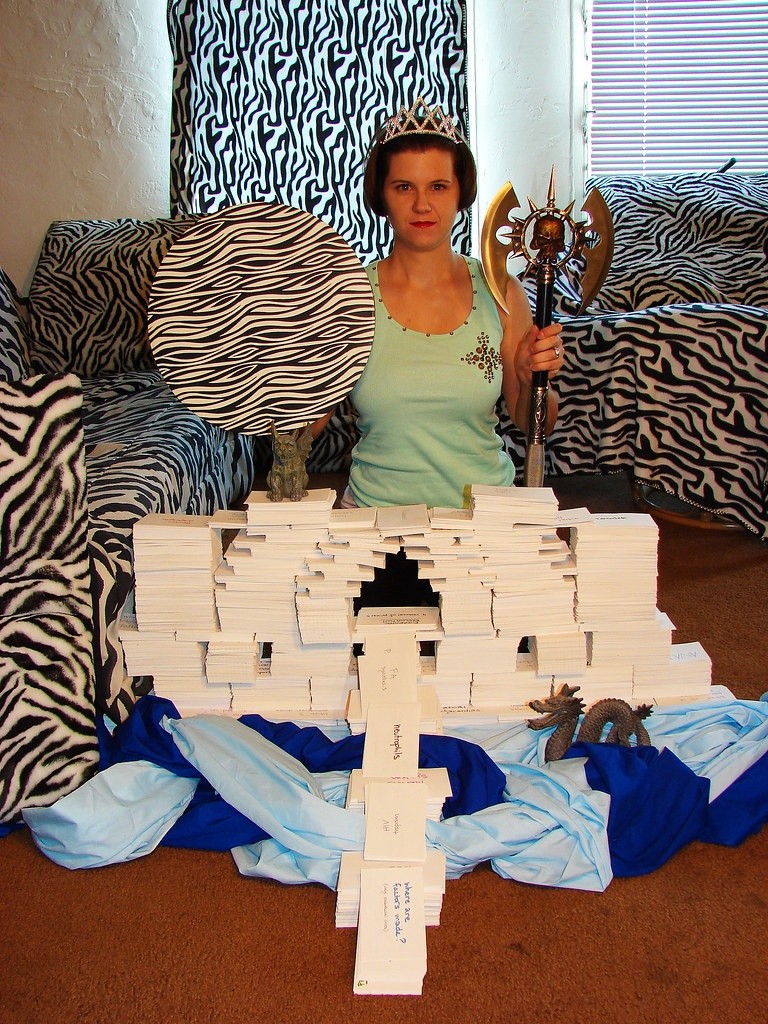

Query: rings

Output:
[552,346,562,358]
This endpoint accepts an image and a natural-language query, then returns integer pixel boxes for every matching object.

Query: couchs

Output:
[0,167,768,827]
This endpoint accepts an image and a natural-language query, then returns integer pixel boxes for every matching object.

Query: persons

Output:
[311,97,569,656]
